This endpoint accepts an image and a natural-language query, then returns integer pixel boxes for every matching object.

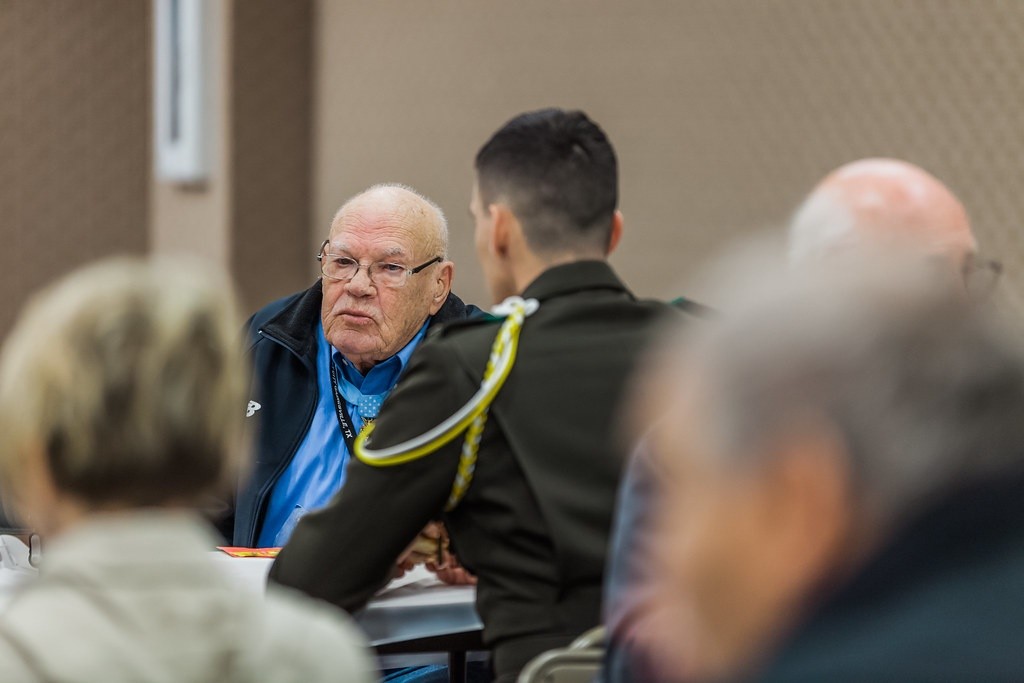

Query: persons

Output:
[0,255,383,683]
[262,107,715,683]
[205,182,494,553]
[604,154,1024,683]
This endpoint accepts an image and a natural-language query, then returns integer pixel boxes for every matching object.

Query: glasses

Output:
[317,239,444,287]
[965,252,1003,307]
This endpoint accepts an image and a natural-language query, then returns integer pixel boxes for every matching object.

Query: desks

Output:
[223,551,494,683]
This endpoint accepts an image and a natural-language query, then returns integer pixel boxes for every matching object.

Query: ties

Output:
[334,365,392,417]
